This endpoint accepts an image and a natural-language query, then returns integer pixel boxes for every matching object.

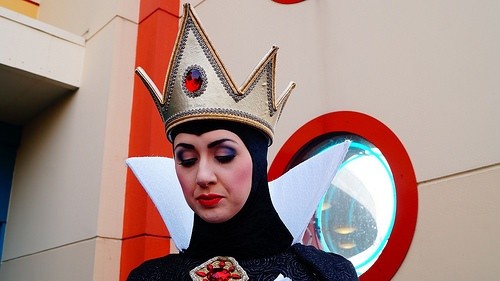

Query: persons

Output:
[124,120,359,281]
